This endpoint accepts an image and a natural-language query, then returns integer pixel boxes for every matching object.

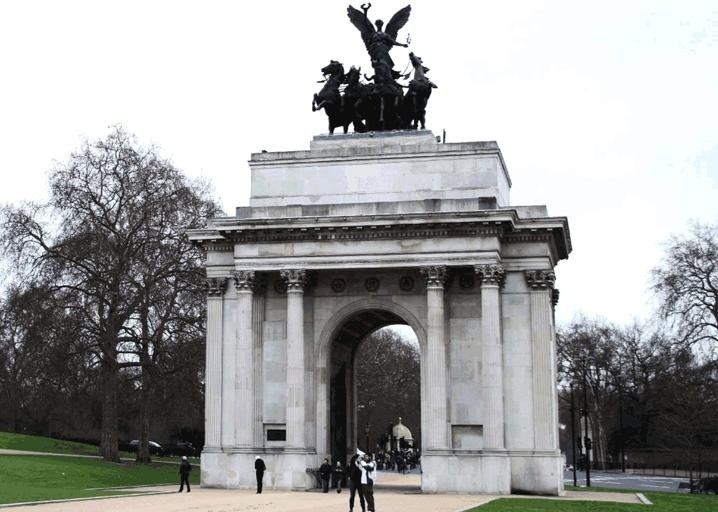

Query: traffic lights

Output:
[577,437,581,449]
[387,423,393,435]
[584,436,588,447]
[400,438,405,448]
[394,435,397,442]
[587,439,591,449]
[580,408,583,417]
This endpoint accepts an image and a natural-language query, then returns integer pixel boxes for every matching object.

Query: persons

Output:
[255,455,266,493]
[319,457,332,493]
[333,460,344,493]
[347,453,366,512]
[367,18,408,77]
[355,452,377,512]
[373,444,421,474]
[178,455,192,492]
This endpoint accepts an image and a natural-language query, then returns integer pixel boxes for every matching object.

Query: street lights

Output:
[363,422,372,454]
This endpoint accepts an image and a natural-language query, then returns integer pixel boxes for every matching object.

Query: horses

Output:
[405,52,438,130]
[342,66,381,134]
[372,58,406,130]
[311,60,353,136]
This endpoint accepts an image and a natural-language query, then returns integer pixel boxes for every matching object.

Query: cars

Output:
[694,477,717,496]
[123,438,166,457]
